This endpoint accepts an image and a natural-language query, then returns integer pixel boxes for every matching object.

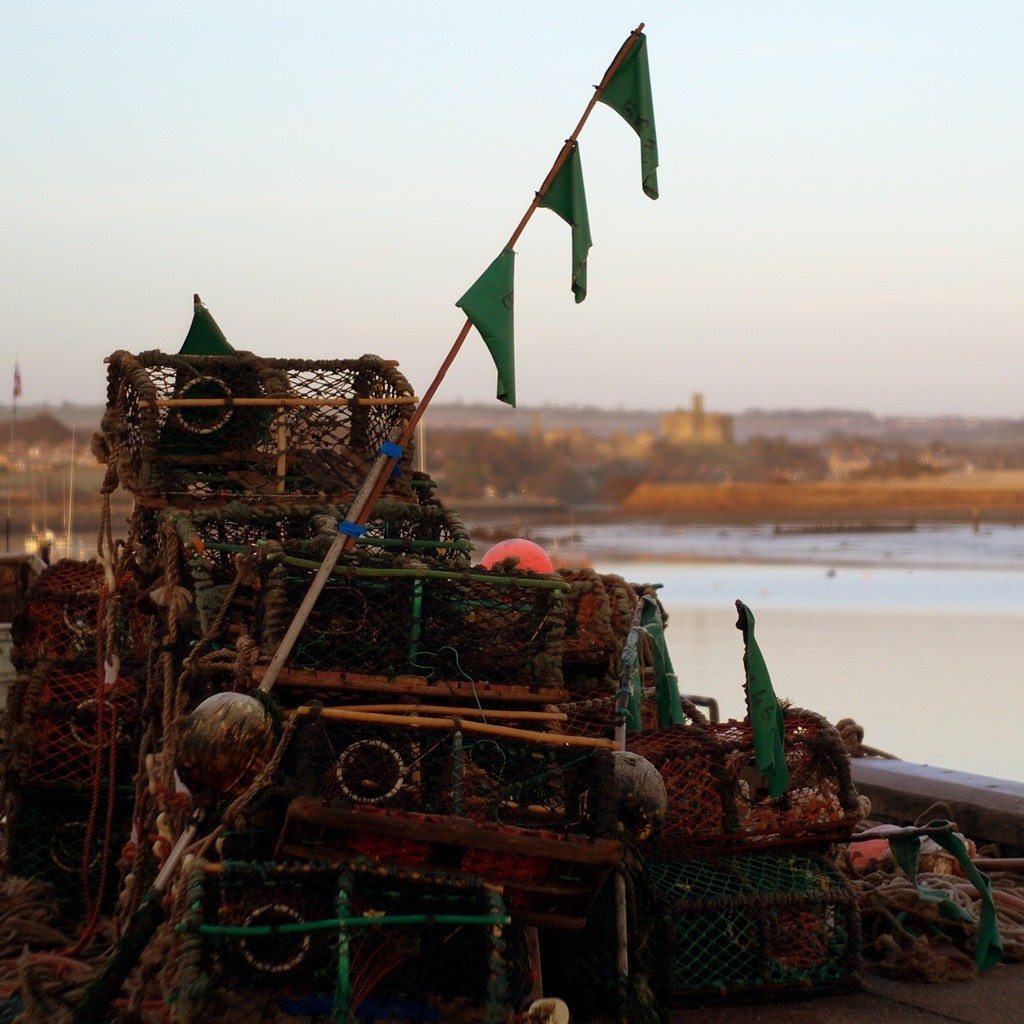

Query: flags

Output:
[735,600,789,799]
[160,304,270,449]
[596,30,662,199]
[455,246,517,410]
[14,365,23,398]
[535,140,592,303]
